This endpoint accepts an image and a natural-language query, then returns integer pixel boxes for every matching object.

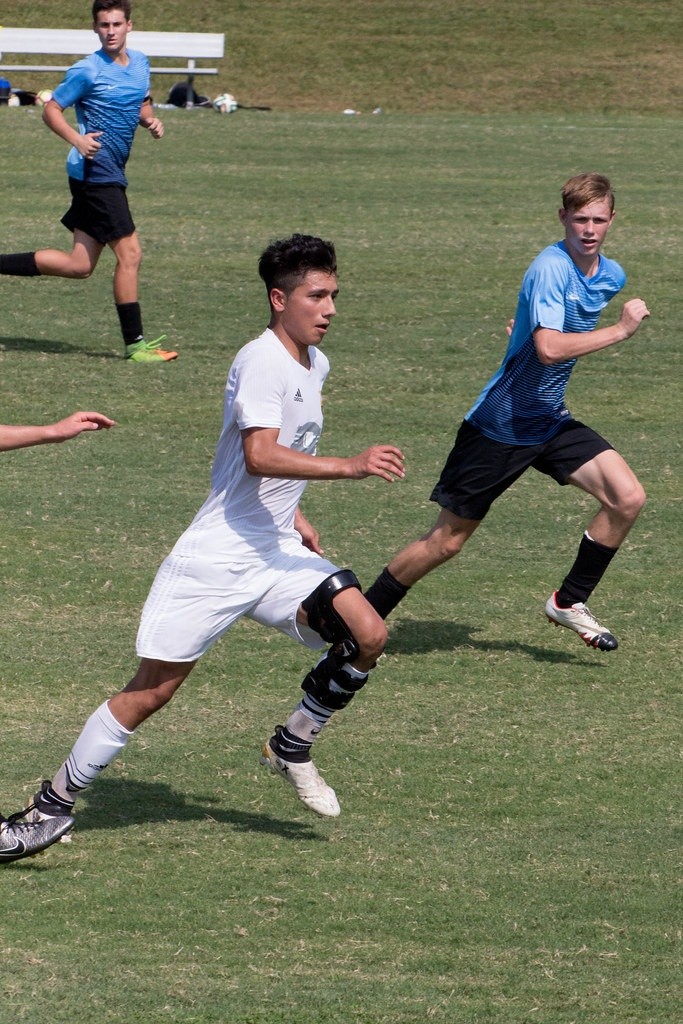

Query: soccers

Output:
[211,92,238,114]
[34,89,54,107]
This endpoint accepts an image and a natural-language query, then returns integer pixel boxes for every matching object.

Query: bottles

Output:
[344,109,362,115]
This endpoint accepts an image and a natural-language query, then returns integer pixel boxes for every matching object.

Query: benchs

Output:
[0,28,224,109]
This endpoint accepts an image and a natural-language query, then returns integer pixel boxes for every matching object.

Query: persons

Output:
[367,169,648,657]
[0,233,408,860]
[0,0,182,363]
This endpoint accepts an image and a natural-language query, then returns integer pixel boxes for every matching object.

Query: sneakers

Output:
[123,335,178,364]
[22,780,74,844]
[259,724,341,818]
[0,802,75,864]
[544,590,619,652]
